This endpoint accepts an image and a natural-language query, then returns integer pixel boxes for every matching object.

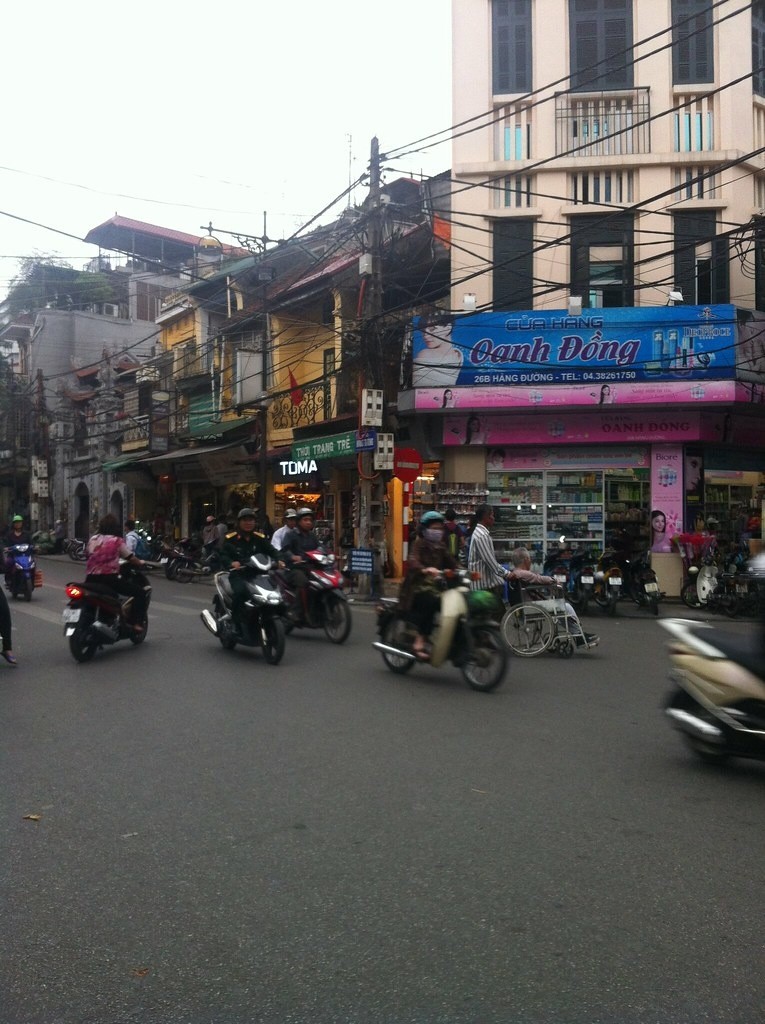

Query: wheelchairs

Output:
[498,576,601,660]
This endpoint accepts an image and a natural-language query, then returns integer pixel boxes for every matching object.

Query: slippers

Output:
[412,649,429,660]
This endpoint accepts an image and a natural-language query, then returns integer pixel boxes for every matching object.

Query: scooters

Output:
[539,531,765,617]
[64,536,89,562]
[656,615,765,767]
[4,542,40,602]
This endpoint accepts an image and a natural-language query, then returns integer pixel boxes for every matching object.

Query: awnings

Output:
[136,436,253,462]
[178,416,257,437]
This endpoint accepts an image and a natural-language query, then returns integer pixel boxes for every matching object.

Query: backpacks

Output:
[131,534,149,560]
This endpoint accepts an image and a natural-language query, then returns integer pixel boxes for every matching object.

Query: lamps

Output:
[567,296,582,315]
[666,291,684,306]
[463,292,476,312]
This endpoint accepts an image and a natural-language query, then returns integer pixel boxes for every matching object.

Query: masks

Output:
[423,529,442,542]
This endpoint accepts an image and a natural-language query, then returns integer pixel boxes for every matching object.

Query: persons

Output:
[0,515,36,665]
[84,513,147,632]
[224,508,288,634]
[685,447,704,492]
[50,520,65,554]
[124,520,142,556]
[202,513,228,558]
[270,509,298,550]
[443,509,465,563]
[692,511,707,534]
[397,510,481,660]
[450,415,486,445]
[744,383,761,403]
[412,315,463,387]
[510,548,600,648]
[651,511,671,553]
[714,414,735,444]
[486,449,505,469]
[433,388,456,408]
[468,503,515,623]
[281,508,340,628]
[590,385,615,404]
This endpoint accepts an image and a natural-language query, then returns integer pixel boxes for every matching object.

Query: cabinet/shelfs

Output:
[276,493,322,526]
[413,472,754,577]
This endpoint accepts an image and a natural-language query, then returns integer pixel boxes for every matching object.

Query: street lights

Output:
[197,210,288,526]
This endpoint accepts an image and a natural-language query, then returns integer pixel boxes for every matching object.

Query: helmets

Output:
[238,508,257,519]
[420,511,442,523]
[13,514,22,522]
[297,508,314,517]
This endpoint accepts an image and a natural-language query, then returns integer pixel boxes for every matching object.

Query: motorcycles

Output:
[370,566,512,692]
[62,557,151,663]
[269,538,355,645]
[132,519,224,583]
[200,546,294,665]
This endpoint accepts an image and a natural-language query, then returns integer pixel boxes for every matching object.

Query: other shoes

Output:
[577,632,598,644]
[127,624,143,633]
[232,621,243,639]
[575,641,599,649]
[1,650,17,665]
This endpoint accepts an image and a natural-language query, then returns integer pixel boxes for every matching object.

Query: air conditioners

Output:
[101,302,118,316]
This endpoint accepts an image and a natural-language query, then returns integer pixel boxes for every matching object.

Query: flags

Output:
[289,370,304,406]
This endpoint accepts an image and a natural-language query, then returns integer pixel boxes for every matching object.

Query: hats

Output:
[707,518,720,524]
[284,508,297,518]
[207,516,217,522]
